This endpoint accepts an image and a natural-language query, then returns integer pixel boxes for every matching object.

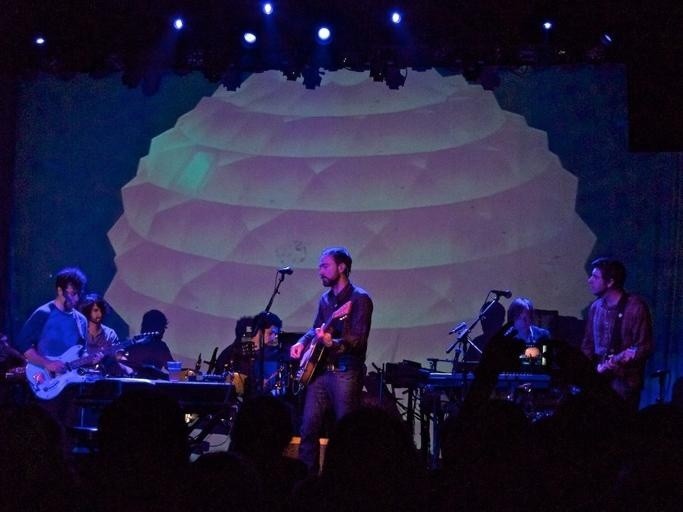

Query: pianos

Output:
[11,376,238,409]
[426,372,551,390]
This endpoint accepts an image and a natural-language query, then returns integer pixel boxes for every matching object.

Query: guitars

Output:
[26,331,160,401]
[297,301,353,384]
[523,348,637,422]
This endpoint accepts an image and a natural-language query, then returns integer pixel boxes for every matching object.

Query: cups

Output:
[167,361,182,382]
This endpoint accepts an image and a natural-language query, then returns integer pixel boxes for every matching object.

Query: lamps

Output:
[219,57,505,92]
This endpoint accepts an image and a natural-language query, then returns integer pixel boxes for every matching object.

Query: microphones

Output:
[453,322,466,333]
[490,289,512,298]
[651,369,670,377]
[275,267,294,275]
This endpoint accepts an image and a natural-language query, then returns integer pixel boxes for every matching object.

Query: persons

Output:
[0,389,683,511]
[506,256,652,411]
[232,248,374,474]
[2,266,132,476]
